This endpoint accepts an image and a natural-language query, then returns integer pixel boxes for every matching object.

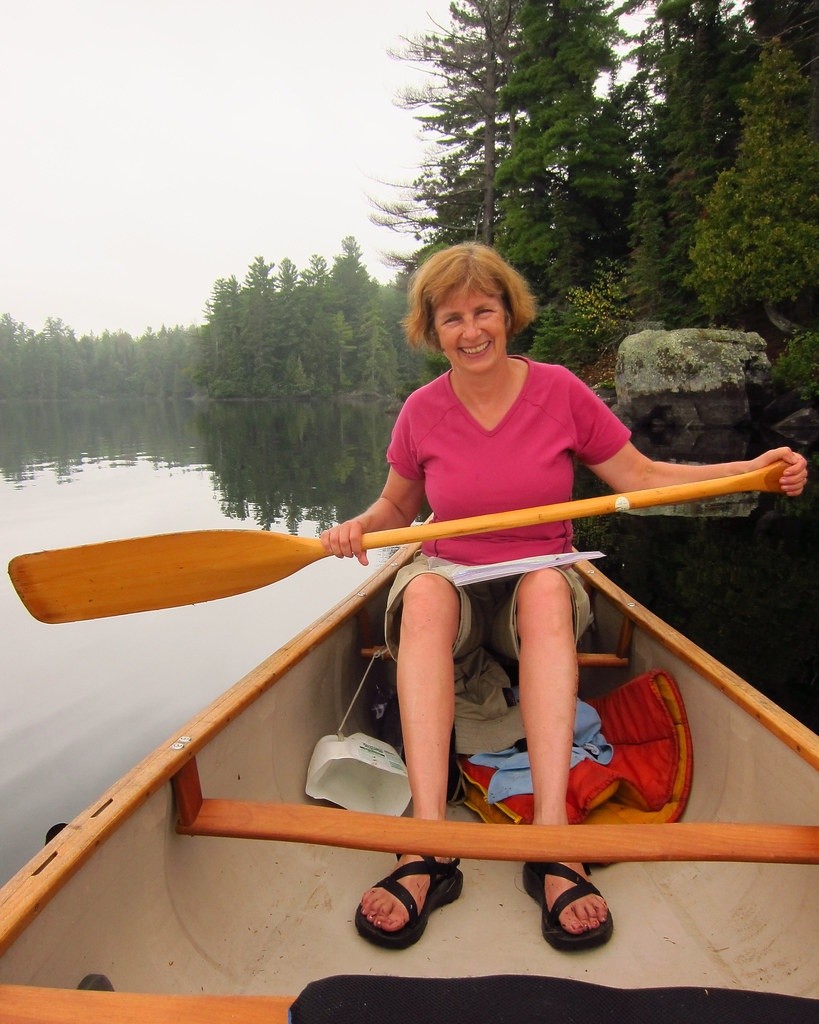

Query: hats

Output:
[454,646,526,755]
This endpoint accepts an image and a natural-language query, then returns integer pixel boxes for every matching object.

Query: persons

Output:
[323,242,807,954]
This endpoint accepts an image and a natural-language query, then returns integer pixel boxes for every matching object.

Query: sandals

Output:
[355,853,464,951]
[523,861,613,953]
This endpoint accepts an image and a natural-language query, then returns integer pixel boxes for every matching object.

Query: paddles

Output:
[6,459,793,628]
